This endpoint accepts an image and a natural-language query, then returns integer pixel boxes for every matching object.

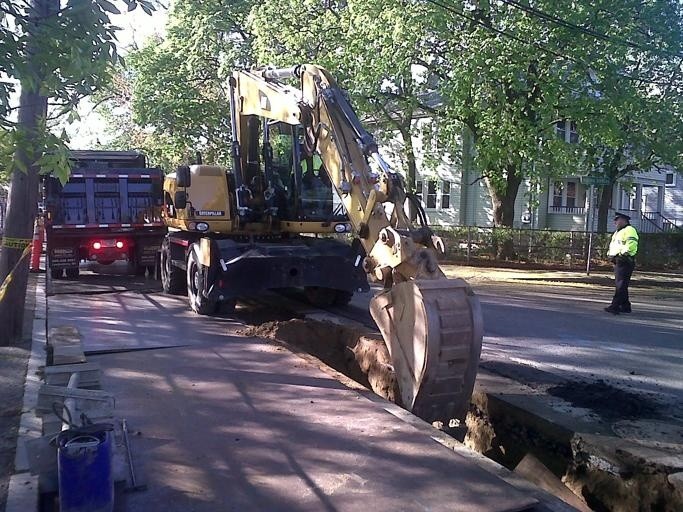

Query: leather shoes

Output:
[603,307,619,315]
[619,308,631,313]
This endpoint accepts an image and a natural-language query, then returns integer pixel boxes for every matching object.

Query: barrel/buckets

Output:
[57,425,115,511]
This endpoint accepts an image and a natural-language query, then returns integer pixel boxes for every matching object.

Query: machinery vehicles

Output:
[159,63,484,425]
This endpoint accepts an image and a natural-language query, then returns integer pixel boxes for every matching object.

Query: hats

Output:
[613,211,631,222]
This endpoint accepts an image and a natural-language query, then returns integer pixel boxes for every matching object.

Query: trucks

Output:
[43,149,165,277]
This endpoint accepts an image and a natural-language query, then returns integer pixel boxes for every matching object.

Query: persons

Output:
[603,212,640,314]
